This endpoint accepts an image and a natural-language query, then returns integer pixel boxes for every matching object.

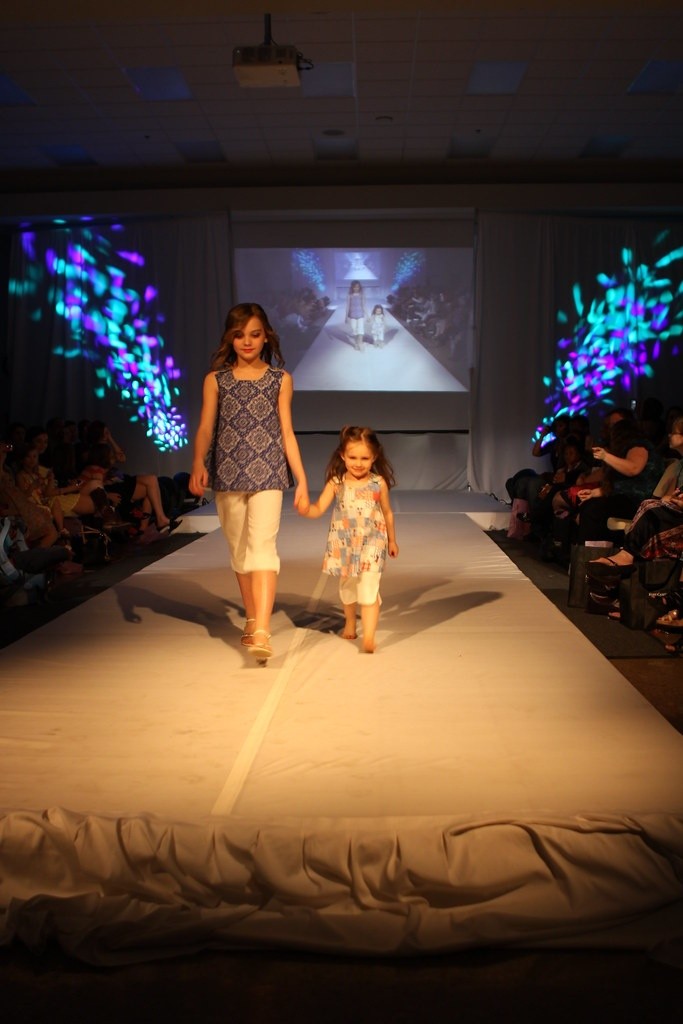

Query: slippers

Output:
[157,519,182,534]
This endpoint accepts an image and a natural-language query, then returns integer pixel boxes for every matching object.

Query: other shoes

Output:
[656,609,683,627]
[664,637,683,656]
[647,590,683,613]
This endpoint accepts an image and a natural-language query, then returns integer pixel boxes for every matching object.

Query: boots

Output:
[91,487,131,530]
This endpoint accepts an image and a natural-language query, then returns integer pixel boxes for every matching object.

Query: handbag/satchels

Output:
[75,524,113,570]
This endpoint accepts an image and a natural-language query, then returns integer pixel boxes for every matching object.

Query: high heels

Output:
[589,591,621,612]
[585,556,637,581]
[241,618,255,646]
[247,630,272,665]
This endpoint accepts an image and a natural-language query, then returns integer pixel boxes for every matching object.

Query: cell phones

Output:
[546,426,557,432]
[0,439,17,452]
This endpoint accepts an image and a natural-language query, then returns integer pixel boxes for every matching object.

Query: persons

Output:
[399,288,470,361]
[368,304,385,349]
[0,419,183,594]
[189,302,310,667]
[257,288,331,340]
[299,427,398,653]
[344,280,367,350]
[506,397,683,652]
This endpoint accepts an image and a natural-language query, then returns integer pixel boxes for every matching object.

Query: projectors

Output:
[231,45,301,89]
